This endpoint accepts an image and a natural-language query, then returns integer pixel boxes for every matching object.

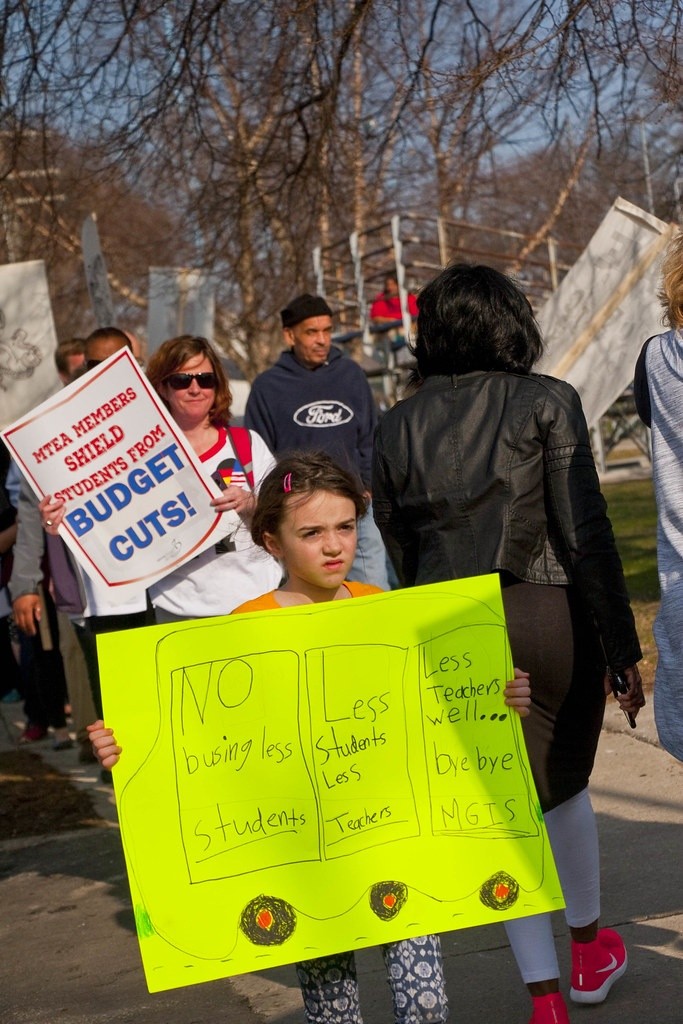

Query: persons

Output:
[370,273,421,402]
[0,292,385,784]
[631,225,683,761]
[368,261,646,1024]
[87,451,531,1024]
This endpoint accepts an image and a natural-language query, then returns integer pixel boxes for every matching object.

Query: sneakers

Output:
[529,992,570,1024]
[571,928,627,1002]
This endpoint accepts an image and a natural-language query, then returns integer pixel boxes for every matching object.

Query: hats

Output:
[281,293,333,327]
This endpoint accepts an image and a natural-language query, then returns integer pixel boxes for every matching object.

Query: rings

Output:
[45,519,53,528]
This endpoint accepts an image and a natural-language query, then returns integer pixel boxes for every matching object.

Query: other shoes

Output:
[78,741,98,765]
[20,722,47,744]
[4,689,23,704]
[100,769,113,786]
[51,734,73,750]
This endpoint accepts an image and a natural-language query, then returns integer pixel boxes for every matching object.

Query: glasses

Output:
[160,373,217,390]
[84,359,102,369]
